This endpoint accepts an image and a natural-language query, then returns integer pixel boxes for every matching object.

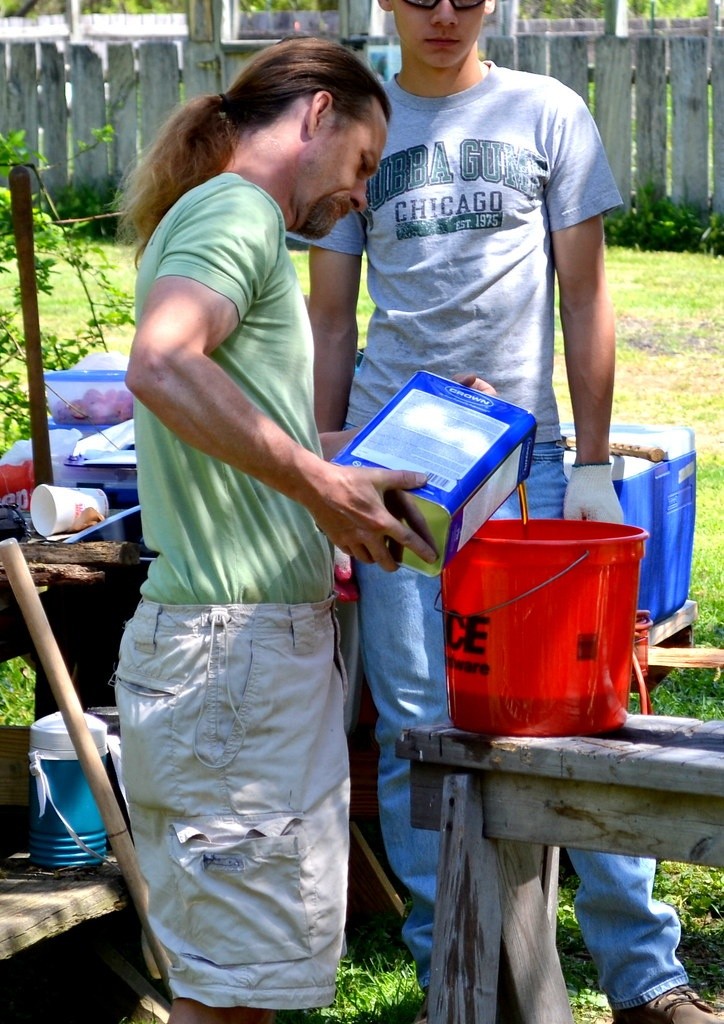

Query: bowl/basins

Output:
[40,369,140,448]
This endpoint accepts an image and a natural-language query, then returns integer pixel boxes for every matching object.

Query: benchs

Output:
[0,850,172,1024]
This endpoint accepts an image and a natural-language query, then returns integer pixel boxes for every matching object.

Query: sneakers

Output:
[611,983,724,1024]
[412,985,475,1024]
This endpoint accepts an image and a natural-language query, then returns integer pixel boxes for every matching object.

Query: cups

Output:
[31,484,109,537]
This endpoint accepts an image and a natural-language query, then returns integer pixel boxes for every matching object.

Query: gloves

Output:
[563,463,624,525]
[332,543,359,603]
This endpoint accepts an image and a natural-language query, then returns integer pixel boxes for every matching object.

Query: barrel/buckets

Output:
[327,370,537,578]
[430,519,648,736]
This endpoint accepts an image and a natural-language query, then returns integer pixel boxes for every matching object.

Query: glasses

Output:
[402,0,484,9]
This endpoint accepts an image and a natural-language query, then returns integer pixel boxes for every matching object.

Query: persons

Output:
[278,0,724,1024]
[106,33,499,1024]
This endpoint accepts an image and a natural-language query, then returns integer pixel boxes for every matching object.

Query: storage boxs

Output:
[560,420,698,623]
[45,370,134,426]
[53,469,140,510]
[48,417,136,482]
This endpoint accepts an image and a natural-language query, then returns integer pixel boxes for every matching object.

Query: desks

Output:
[394,714,724,1024]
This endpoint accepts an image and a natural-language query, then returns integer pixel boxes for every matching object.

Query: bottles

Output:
[29,710,109,866]
